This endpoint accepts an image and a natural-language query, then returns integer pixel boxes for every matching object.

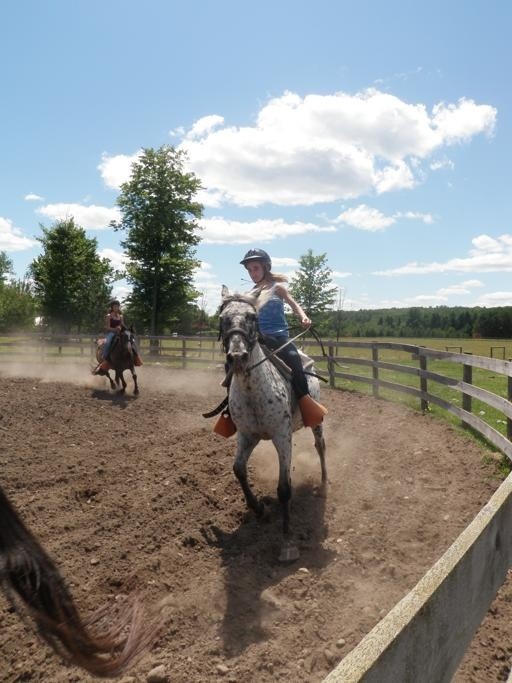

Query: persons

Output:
[100,300,143,371]
[214,248,328,437]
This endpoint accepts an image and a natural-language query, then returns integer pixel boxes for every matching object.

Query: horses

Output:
[88,321,138,394]
[218,284,327,562]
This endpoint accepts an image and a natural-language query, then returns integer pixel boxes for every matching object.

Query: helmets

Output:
[240,249,271,264]
